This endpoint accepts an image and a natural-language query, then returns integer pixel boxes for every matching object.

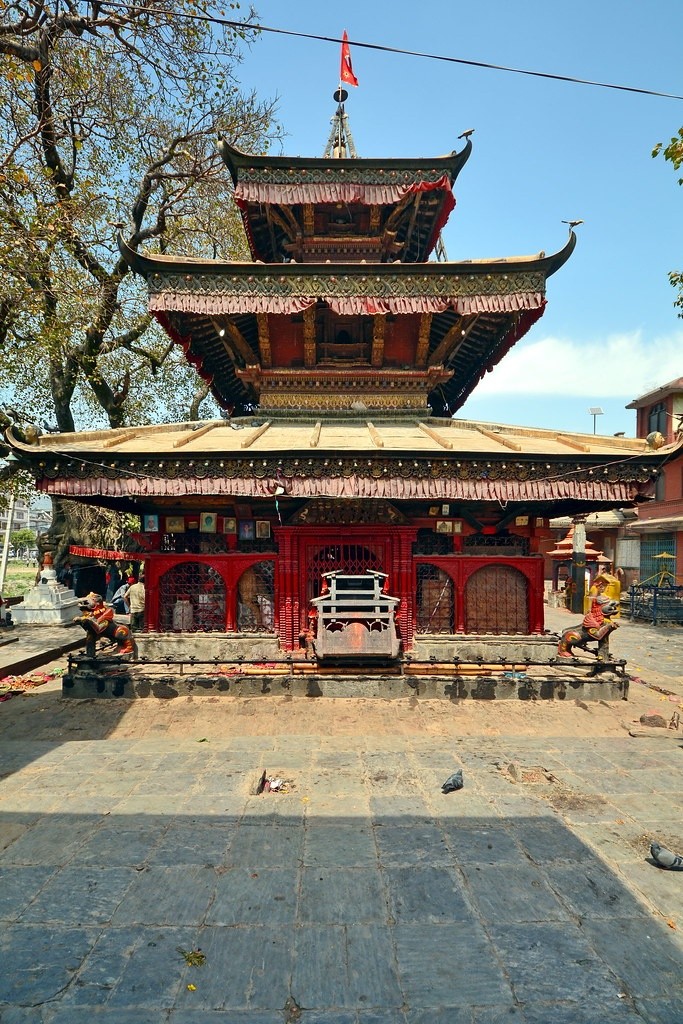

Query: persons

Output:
[111,575,146,630]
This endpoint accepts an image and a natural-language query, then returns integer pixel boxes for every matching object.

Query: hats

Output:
[128,578,136,584]
